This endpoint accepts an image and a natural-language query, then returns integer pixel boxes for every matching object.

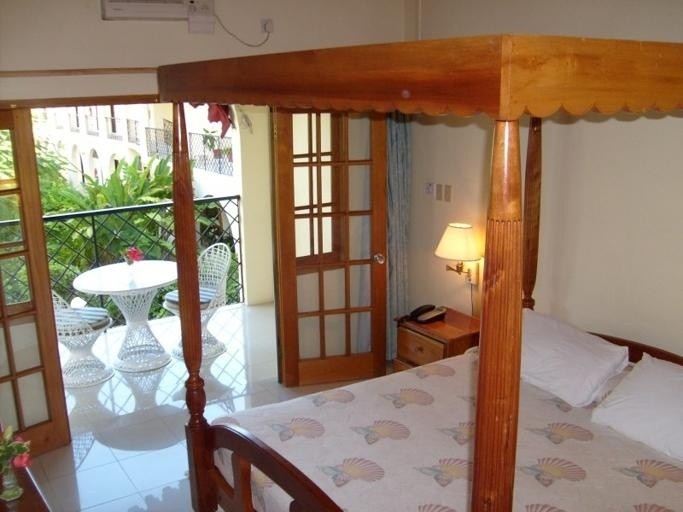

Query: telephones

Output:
[408,301,449,323]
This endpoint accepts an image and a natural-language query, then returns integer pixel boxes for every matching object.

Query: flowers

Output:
[118,246,142,265]
[0,424,31,477]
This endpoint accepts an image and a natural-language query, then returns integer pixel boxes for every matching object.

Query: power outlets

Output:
[435,184,443,201]
[260,18,273,33]
[444,184,451,203]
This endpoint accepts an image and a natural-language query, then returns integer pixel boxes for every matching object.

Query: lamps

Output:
[434,222,482,285]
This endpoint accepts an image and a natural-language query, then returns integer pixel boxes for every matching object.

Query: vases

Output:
[126,259,136,288]
[0,468,52,512]
[0,473,24,502]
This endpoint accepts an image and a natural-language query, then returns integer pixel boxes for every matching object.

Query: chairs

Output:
[163,243,227,360]
[51,289,116,389]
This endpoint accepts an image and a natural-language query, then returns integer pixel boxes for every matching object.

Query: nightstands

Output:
[392,306,480,373]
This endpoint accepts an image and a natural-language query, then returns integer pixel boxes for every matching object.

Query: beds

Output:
[153,315,681,506]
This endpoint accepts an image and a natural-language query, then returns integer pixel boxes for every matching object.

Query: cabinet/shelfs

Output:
[53,466,53,468]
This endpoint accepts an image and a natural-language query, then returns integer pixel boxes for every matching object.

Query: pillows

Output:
[591,352,683,462]
[520,308,629,407]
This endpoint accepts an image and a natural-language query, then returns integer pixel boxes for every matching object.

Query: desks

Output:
[72,260,178,372]
